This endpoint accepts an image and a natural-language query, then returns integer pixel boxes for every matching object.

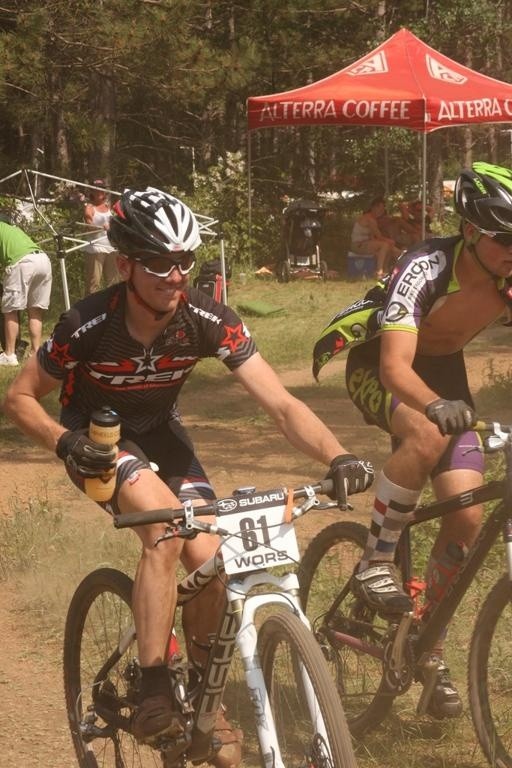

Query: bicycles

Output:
[64,469,372,766]
[292,418,511,767]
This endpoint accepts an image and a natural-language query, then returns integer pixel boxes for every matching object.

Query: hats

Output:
[91,179,107,190]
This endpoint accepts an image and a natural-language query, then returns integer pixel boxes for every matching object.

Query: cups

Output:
[423,544,460,601]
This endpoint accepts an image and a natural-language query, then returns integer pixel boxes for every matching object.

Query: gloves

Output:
[323,451,376,512]
[424,398,480,437]
[55,425,117,482]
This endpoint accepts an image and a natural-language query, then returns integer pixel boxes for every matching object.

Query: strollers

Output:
[275,195,328,286]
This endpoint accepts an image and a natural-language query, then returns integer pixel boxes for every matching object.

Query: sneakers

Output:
[414,655,464,720]
[348,556,416,615]
[194,706,245,767]
[1,351,19,369]
[131,671,182,752]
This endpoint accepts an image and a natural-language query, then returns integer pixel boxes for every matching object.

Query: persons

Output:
[82,180,118,294]
[349,198,435,281]
[0,220,54,366]
[0,187,377,767]
[313,163,511,720]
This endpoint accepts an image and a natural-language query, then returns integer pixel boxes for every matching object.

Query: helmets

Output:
[105,185,204,262]
[455,161,512,249]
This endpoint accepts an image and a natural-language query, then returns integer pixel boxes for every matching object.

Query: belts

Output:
[28,249,45,255]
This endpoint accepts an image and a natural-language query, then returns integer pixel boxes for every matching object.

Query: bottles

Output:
[84,405,120,501]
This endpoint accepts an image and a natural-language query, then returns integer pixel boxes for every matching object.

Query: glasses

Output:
[463,214,512,250]
[119,248,199,279]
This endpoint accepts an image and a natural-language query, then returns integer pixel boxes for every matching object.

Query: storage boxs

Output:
[347,250,375,277]
